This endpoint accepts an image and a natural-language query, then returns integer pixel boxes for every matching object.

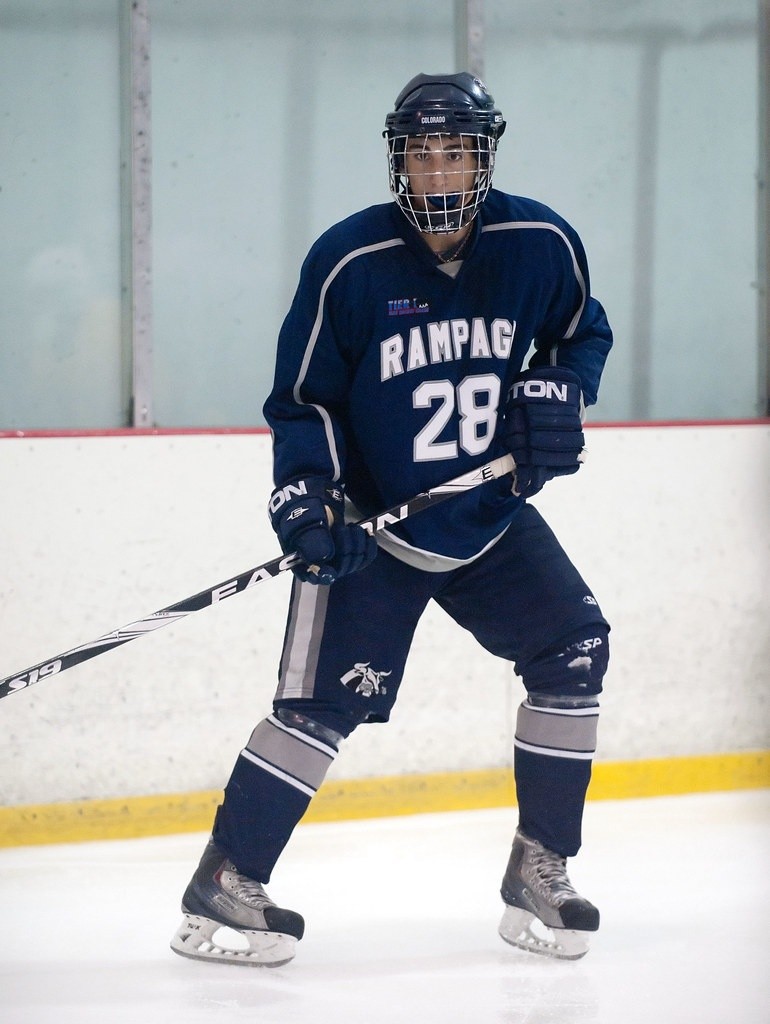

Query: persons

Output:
[171,72,612,963]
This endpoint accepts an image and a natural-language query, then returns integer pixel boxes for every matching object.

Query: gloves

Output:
[266,474,377,583]
[498,382,584,498]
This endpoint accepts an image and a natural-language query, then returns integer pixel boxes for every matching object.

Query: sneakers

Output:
[170,834,304,968]
[498,827,600,960]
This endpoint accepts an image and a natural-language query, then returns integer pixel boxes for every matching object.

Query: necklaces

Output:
[432,222,474,264]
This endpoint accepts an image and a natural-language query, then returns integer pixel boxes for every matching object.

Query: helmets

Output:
[383,73,503,234]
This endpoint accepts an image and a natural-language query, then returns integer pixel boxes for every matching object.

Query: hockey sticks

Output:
[0,445,520,700]
